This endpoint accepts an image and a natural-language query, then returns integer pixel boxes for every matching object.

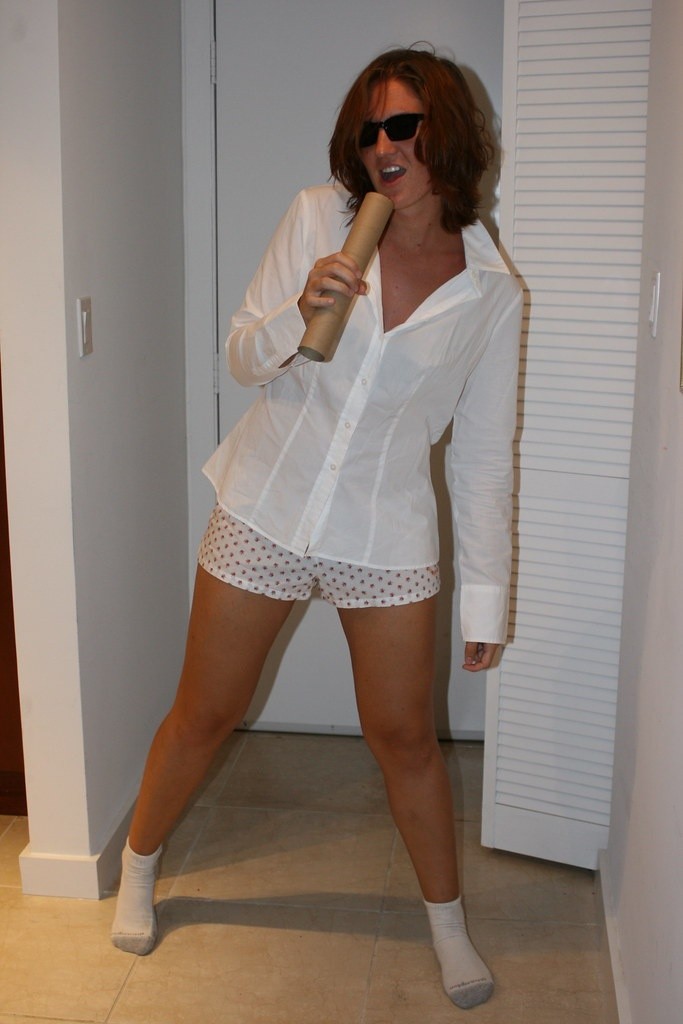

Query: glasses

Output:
[357,112,426,148]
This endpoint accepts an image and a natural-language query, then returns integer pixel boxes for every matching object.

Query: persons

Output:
[110,46,522,1009]
